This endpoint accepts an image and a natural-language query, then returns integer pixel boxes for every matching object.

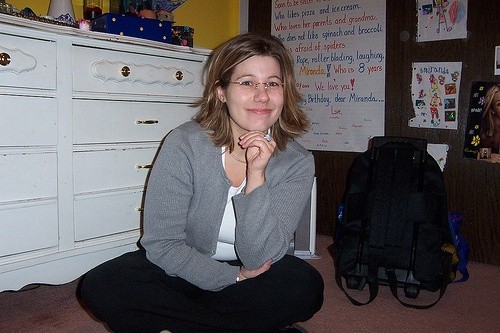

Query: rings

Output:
[264,134,272,141]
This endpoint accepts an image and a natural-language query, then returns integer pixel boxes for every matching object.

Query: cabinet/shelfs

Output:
[0,12,217,291]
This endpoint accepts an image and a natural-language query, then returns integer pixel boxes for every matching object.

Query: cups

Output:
[83,0,104,20]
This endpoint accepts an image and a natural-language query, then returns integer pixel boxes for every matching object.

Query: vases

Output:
[45,0,76,23]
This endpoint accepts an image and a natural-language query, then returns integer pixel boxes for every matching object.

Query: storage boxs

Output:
[89,13,195,47]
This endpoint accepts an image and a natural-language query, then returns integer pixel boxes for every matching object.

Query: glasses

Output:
[229,80,284,92]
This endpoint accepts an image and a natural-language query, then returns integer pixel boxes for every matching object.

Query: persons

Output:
[75,32,324,333]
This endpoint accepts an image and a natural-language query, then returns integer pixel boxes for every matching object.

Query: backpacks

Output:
[333,137,458,310]
